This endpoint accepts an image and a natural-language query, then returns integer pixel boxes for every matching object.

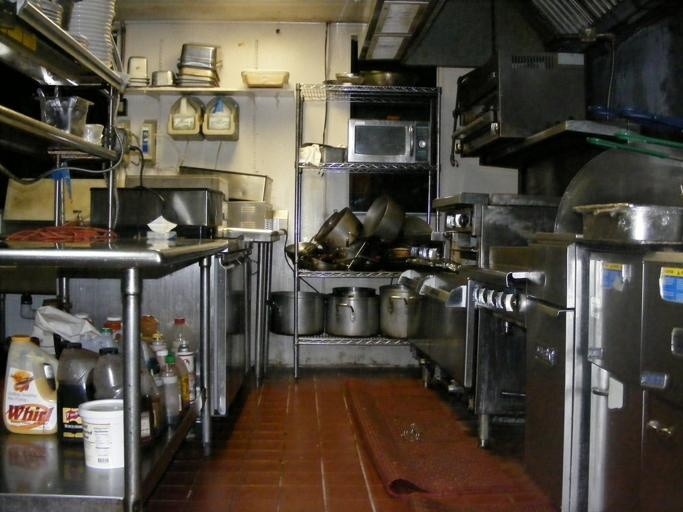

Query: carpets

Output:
[342,377,521,499]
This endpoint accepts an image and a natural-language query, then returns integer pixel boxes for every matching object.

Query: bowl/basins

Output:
[336,72,365,86]
[371,70,401,86]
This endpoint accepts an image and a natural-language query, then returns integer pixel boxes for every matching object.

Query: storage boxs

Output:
[226,201,273,231]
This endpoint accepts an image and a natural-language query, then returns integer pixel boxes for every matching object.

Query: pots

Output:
[264,290,325,337]
[284,193,431,271]
[380,282,417,340]
[326,287,379,336]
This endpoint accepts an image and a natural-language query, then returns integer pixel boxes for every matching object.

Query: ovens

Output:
[67,236,250,419]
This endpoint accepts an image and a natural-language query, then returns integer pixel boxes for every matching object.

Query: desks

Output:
[0,238,229,512]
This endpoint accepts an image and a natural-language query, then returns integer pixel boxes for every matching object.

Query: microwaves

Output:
[347,117,431,165]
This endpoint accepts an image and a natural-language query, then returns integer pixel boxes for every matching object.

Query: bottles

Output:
[101,318,197,434]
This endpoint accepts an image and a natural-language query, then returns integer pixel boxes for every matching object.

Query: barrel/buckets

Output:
[57,342,99,443]
[78,400,125,471]
[96,345,163,446]
[2,334,60,435]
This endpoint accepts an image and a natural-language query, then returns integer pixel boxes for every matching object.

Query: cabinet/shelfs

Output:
[295,82,442,378]
[0,0,125,246]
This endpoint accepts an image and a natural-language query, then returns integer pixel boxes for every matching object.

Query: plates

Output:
[66,0,116,70]
[37,1,62,28]
